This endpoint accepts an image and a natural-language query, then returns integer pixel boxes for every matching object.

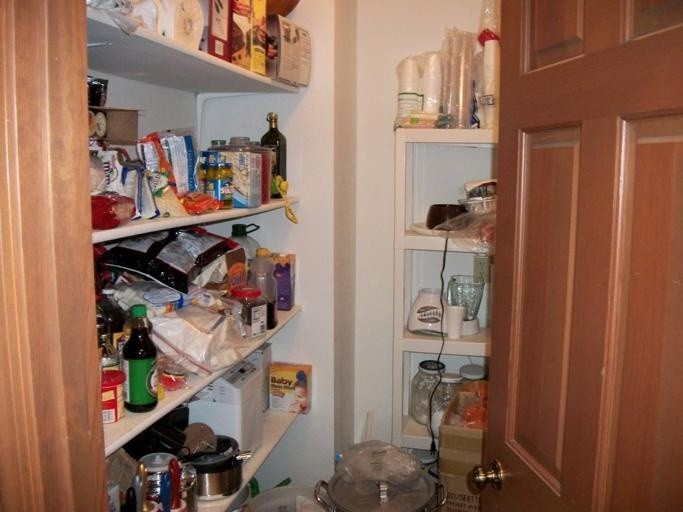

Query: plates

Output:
[409,222,467,237]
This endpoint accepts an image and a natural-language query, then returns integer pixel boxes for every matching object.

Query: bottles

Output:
[410,359,446,426]
[98,288,158,413]
[247,247,279,330]
[220,285,267,345]
[426,372,463,439]
[261,112,287,200]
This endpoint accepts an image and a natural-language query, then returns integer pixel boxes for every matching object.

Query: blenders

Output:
[406,274,485,336]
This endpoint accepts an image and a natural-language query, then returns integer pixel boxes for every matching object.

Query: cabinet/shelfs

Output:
[392,127,497,510]
[88,1,313,511]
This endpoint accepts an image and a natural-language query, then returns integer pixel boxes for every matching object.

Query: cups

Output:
[445,305,465,341]
[396,31,501,129]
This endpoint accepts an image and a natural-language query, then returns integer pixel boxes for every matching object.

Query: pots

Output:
[313,468,448,512]
[180,434,253,502]
